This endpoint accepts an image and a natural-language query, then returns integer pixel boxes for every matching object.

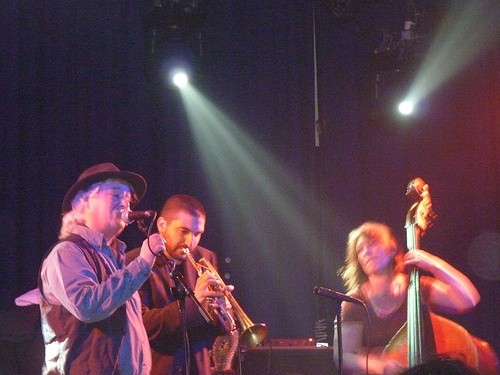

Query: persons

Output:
[124,193,235,375]
[39,163,166,375]
[333,222,500,375]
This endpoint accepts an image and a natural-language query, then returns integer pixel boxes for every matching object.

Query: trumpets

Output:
[182,245,268,349]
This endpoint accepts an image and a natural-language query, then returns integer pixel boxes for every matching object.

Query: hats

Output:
[62,163,147,215]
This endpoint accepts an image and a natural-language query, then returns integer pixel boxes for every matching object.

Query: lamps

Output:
[142,0,208,101]
[364,20,456,137]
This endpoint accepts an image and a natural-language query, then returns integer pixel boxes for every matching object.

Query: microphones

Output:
[120,209,155,224]
[312,286,365,307]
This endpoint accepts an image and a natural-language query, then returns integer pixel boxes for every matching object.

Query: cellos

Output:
[380,177,500,375]
